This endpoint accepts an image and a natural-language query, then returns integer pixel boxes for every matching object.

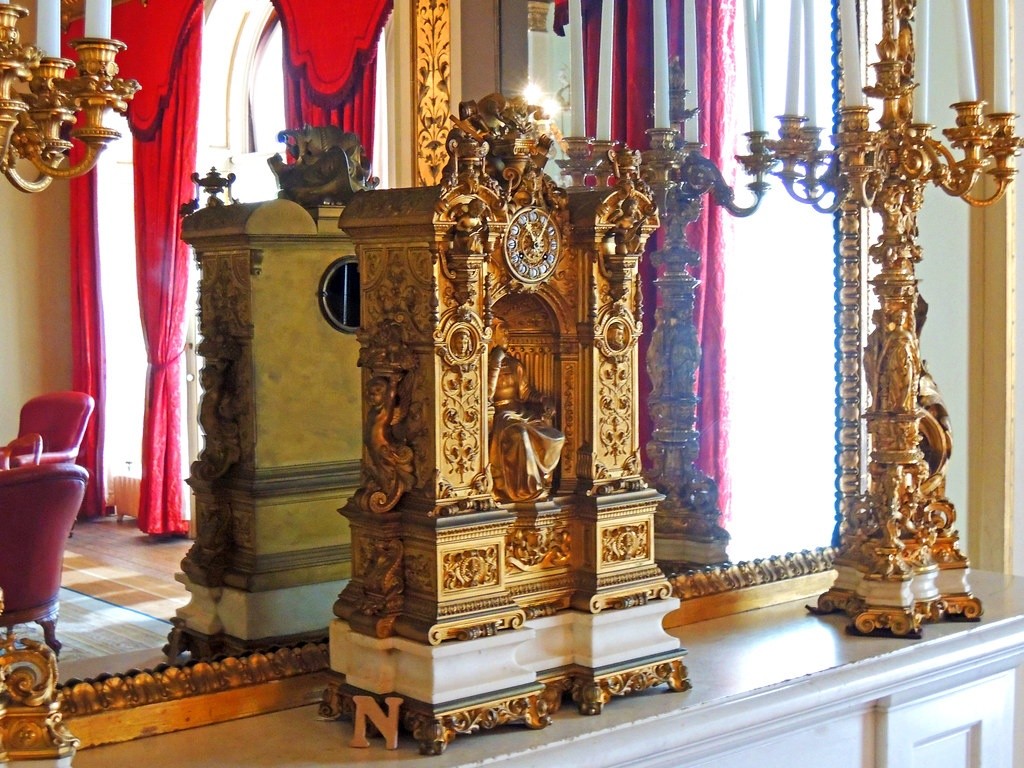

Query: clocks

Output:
[317,94,691,756]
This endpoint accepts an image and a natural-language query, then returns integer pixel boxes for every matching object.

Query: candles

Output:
[37,0,61,58]
[653,0,670,129]
[992,1,1010,114]
[596,0,615,140]
[840,0,862,108]
[683,0,699,142]
[785,1,802,117]
[569,1,586,137]
[804,1,816,128]
[84,0,111,39]
[914,0,930,125]
[746,1,764,129]
[954,1,978,103]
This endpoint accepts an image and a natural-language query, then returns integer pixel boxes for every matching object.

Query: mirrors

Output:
[0,0,867,751]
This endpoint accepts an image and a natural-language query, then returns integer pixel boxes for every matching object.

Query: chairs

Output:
[0,391,95,469]
[0,463,89,656]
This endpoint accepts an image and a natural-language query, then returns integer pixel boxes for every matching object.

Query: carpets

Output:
[1,586,193,686]
